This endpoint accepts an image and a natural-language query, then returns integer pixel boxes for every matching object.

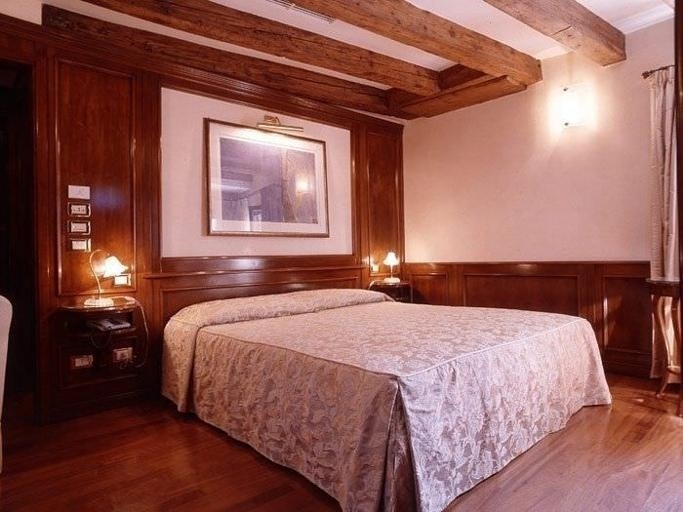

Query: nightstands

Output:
[371,282,412,304]
[56,302,147,417]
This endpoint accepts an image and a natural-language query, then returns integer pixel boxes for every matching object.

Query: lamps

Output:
[85,248,128,307]
[256,115,304,132]
[383,251,402,285]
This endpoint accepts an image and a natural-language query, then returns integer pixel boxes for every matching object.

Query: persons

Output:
[278,176,299,223]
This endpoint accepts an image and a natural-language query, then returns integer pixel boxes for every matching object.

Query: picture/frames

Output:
[204,117,330,237]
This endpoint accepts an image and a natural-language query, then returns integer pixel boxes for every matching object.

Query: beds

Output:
[172,287,593,512]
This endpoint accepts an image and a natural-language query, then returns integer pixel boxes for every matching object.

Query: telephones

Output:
[87,320,130,331]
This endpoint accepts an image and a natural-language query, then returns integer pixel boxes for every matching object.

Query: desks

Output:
[645,276,683,418]
